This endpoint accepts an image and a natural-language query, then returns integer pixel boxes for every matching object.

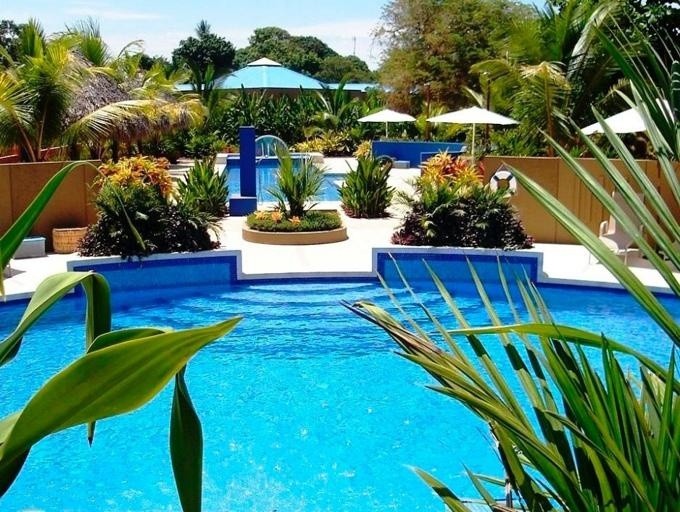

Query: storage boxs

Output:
[12,236,46,259]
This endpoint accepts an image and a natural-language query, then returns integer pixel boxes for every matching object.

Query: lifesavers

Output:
[489,171,517,198]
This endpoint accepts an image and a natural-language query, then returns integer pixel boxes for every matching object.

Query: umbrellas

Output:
[581,98,675,136]
[426,105,522,162]
[208,58,329,90]
[358,109,417,137]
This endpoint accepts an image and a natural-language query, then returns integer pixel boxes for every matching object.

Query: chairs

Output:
[588,191,644,266]
